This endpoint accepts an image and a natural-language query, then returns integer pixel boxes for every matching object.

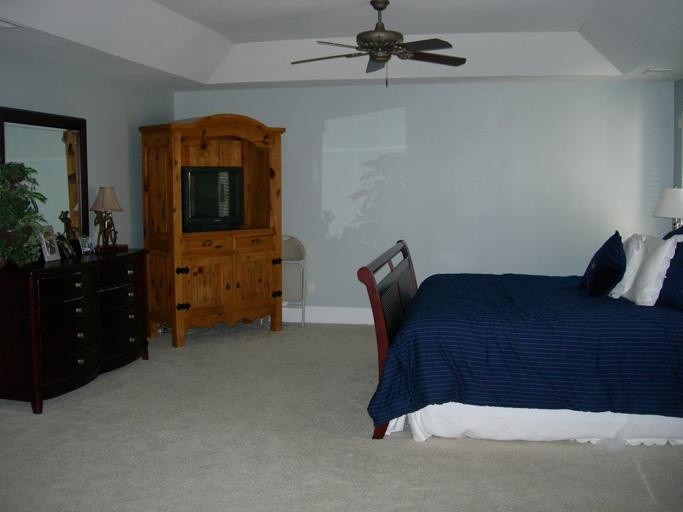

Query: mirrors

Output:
[0,105,90,247]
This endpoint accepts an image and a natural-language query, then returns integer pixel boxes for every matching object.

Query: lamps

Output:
[649,185,683,230]
[87,185,130,254]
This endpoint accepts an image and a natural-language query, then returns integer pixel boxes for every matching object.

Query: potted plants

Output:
[0,160,49,269]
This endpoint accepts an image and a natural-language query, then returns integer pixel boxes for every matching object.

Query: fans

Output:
[290,1,469,75]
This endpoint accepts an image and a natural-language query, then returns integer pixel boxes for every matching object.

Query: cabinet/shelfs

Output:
[136,111,289,350]
[1,247,151,416]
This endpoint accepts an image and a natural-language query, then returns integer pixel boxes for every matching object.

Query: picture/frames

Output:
[37,223,62,263]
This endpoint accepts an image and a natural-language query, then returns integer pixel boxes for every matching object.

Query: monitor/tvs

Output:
[181,165,245,231]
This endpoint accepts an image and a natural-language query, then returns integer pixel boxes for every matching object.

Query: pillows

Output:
[621,234,683,308]
[657,225,683,309]
[606,232,648,300]
[575,229,627,298]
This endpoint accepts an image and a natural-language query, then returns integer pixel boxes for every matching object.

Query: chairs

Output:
[259,235,307,327]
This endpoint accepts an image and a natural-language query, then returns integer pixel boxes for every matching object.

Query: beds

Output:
[354,238,682,448]
